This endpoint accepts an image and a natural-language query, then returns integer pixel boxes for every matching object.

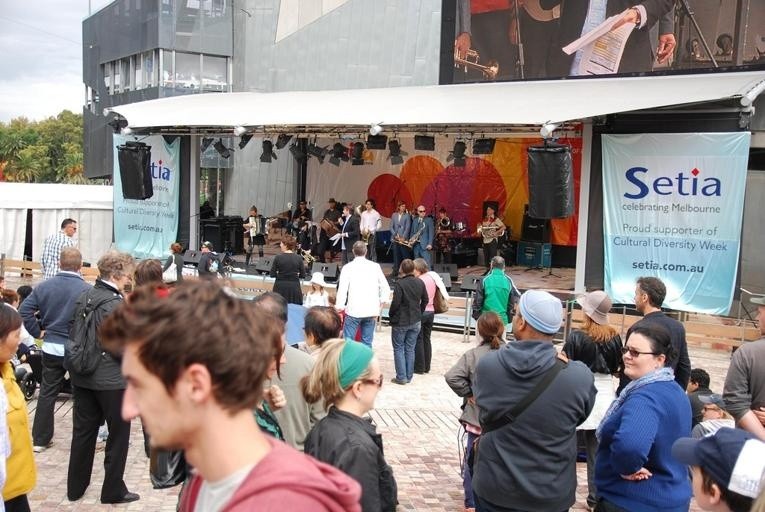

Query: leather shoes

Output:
[123,492,141,502]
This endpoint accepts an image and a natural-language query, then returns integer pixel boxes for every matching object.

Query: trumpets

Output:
[454,49,499,80]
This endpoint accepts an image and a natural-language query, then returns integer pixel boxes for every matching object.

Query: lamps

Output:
[198,134,498,167]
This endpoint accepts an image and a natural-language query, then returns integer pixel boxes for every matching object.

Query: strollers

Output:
[15,343,74,407]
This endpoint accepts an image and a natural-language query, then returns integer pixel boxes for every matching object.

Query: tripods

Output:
[524,226,544,272]
[542,232,562,279]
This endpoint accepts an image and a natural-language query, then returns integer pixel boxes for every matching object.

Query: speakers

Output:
[183,216,247,268]
[527,145,574,219]
[310,262,340,281]
[516,240,552,267]
[461,274,485,292]
[482,201,499,219]
[254,256,276,275]
[520,204,552,243]
[437,273,452,291]
[117,141,153,200]
[431,263,458,281]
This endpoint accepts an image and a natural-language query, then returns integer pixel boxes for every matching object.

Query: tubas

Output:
[477,223,505,237]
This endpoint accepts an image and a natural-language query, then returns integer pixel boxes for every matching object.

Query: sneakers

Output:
[391,378,407,385]
[33,438,53,453]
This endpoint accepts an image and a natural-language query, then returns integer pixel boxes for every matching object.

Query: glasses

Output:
[703,406,715,412]
[620,348,659,358]
[419,211,426,213]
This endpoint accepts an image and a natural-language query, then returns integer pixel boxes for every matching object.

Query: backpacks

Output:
[162,263,178,283]
[206,255,220,273]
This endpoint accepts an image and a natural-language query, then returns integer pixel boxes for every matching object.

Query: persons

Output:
[454,0,765,82]
[1,197,765,512]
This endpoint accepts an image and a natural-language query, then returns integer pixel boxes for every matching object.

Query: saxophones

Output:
[408,222,426,249]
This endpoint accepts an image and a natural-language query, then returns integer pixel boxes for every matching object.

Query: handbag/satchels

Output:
[434,286,449,314]
[62,286,122,377]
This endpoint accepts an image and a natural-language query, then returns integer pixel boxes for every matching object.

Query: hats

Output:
[576,289,613,327]
[309,272,327,287]
[326,198,337,204]
[697,393,725,411]
[200,241,214,252]
[670,426,765,499]
[518,287,563,336]
